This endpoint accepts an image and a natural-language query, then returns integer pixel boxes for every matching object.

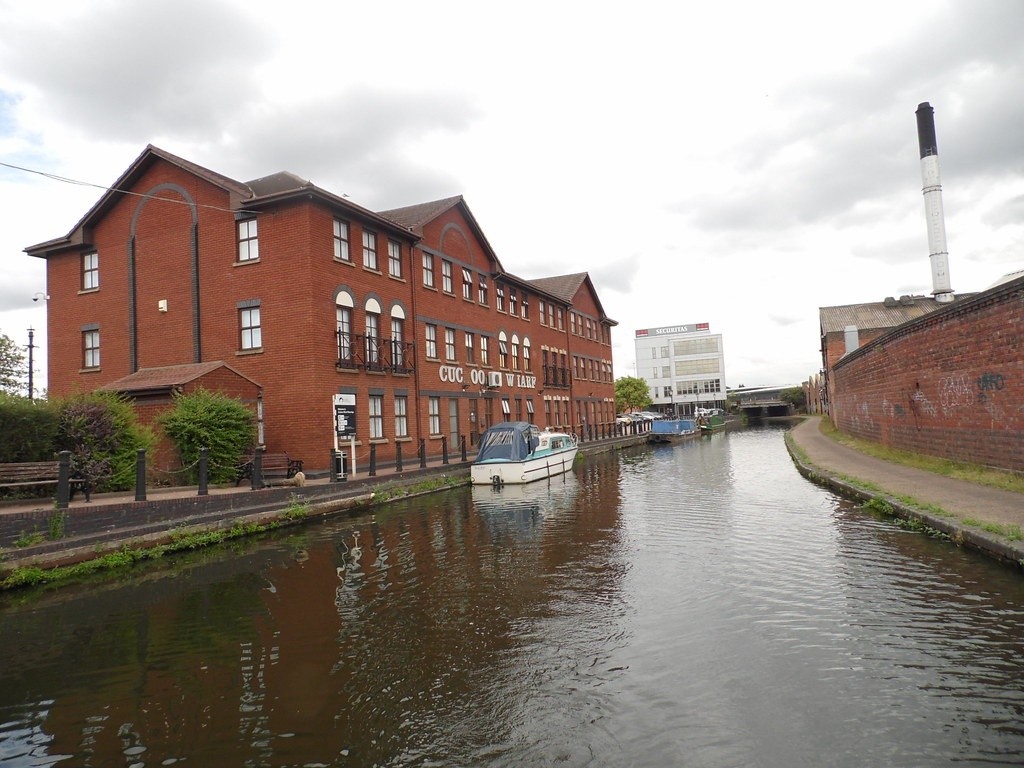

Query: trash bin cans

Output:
[330,449,348,482]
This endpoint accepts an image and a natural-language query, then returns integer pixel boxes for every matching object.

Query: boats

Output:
[470,422,578,485]
[696,410,727,430]
[648,420,702,441]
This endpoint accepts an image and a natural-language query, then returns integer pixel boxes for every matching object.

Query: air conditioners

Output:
[488,372,503,387]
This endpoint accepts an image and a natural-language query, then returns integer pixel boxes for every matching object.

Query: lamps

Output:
[462,384,470,391]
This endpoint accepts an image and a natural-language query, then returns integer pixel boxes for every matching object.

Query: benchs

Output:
[0,460,92,503]
[233,451,305,487]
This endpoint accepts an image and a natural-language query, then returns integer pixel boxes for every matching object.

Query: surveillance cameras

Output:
[32,295,38,301]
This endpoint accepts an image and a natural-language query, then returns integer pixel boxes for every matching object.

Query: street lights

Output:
[696,393,700,407]
[713,393,716,410]
[669,389,674,415]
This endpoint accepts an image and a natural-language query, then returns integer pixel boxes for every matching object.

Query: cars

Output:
[615,411,668,427]
